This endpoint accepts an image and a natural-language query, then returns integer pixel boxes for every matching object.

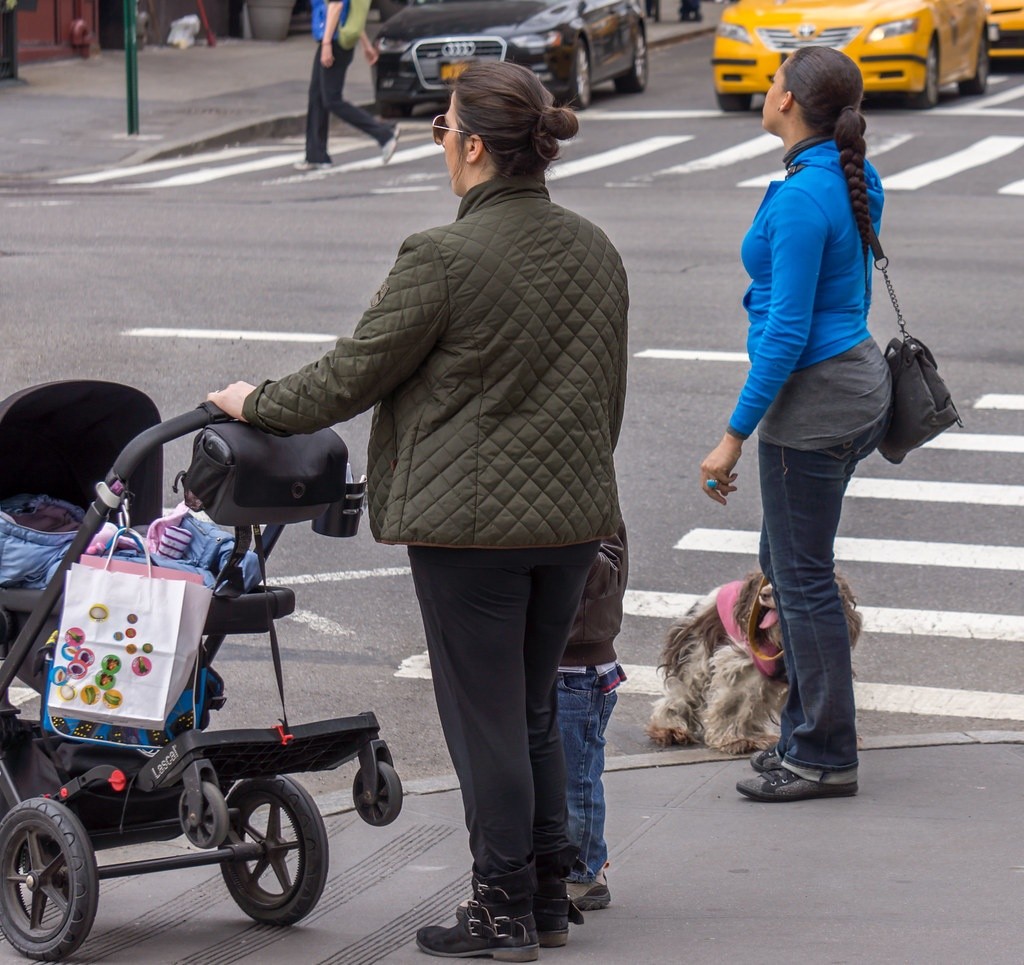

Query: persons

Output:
[700,46,895,803]
[207,63,630,961]
[294,0,401,172]
[642,0,703,24]
[554,496,629,910]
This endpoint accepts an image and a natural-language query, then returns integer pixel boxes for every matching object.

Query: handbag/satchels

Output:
[49,525,214,728]
[872,336,966,466]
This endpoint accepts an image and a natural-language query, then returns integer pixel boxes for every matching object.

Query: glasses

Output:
[431,114,494,156]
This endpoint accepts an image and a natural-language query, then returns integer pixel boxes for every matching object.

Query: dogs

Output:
[646,571,863,755]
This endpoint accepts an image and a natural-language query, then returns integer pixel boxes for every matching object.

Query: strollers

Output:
[0,377,405,964]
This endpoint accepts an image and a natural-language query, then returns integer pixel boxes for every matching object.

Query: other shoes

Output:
[381,125,400,163]
[294,162,332,169]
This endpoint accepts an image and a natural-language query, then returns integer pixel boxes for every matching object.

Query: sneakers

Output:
[735,766,858,801]
[566,863,610,910]
[748,745,785,773]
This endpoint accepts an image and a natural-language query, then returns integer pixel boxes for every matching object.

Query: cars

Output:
[711,0,1024,113]
[368,1,649,121]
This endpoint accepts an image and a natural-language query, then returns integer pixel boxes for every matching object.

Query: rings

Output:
[215,390,221,393]
[707,479,718,490]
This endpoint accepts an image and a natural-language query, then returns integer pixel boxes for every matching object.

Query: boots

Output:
[416,853,540,963]
[534,892,583,946]
